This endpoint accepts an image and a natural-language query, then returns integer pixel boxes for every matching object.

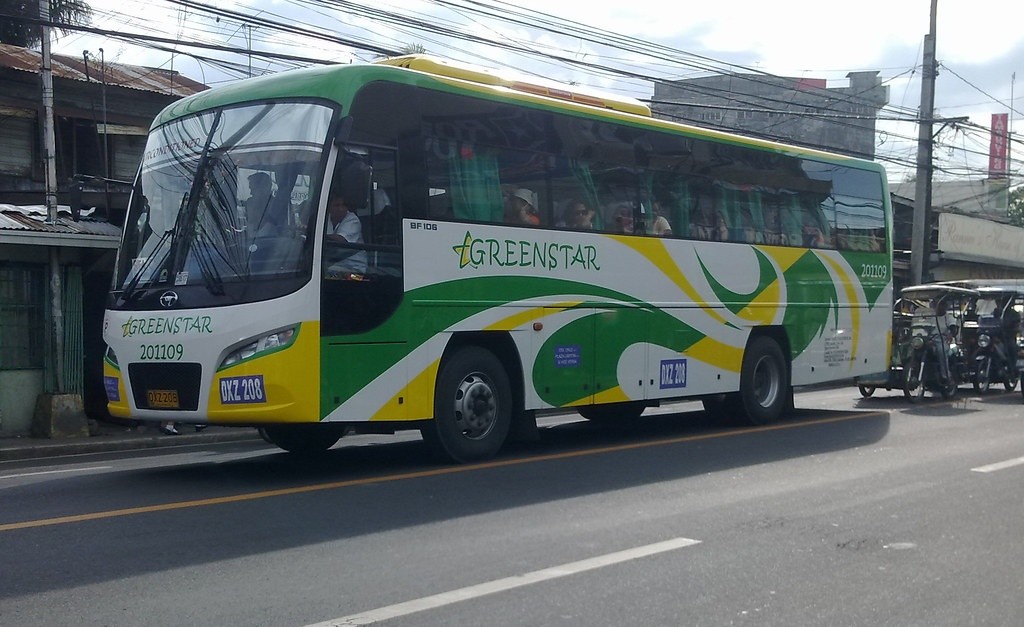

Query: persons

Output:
[921,298,959,394]
[322,195,367,271]
[995,300,1021,369]
[244,169,827,251]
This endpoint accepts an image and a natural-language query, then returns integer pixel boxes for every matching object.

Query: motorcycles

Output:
[856,279,1024,404]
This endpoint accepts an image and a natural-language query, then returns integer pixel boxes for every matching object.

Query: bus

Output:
[69,51,895,465]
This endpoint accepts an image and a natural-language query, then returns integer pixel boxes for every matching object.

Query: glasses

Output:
[575,210,585,216]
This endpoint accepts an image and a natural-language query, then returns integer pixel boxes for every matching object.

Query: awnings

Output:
[0,211,123,248]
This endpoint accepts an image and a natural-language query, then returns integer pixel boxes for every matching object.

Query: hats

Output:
[515,189,538,211]
